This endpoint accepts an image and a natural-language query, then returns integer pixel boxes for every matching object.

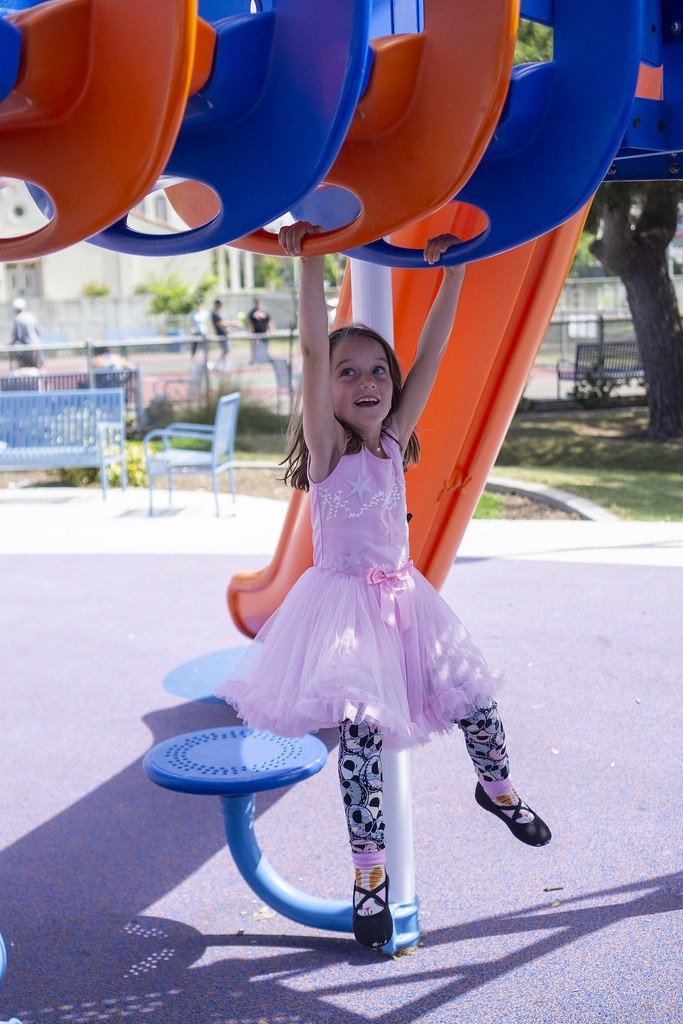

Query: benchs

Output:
[555,339,645,400]
[0,362,146,431]
[0,385,132,500]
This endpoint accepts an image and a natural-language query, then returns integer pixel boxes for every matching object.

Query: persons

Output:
[8,299,45,371]
[248,298,276,366]
[188,299,209,356]
[212,301,240,374]
[217,220,552,949]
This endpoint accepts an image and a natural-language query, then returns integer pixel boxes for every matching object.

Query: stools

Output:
[140,723,354,936]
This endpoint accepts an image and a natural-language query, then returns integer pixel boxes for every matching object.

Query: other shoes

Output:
[353,871,393,948]
[476,781,551,846]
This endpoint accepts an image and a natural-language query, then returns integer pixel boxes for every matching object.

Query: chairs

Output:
[271,358,303,413]
[143,391,241,519]
[152,363,205,420]
